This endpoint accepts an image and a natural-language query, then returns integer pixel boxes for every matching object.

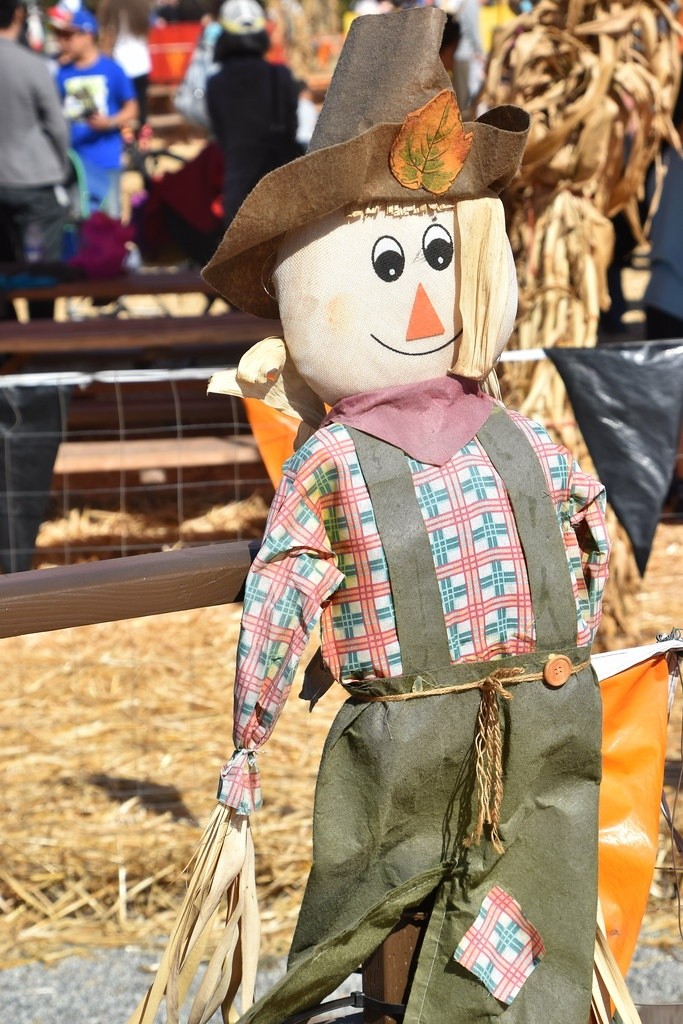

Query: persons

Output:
[0,0,156,321]
[202,2,298,220]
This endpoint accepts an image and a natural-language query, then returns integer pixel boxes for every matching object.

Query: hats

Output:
[217,0,264,35]
[46,0,98,34]
